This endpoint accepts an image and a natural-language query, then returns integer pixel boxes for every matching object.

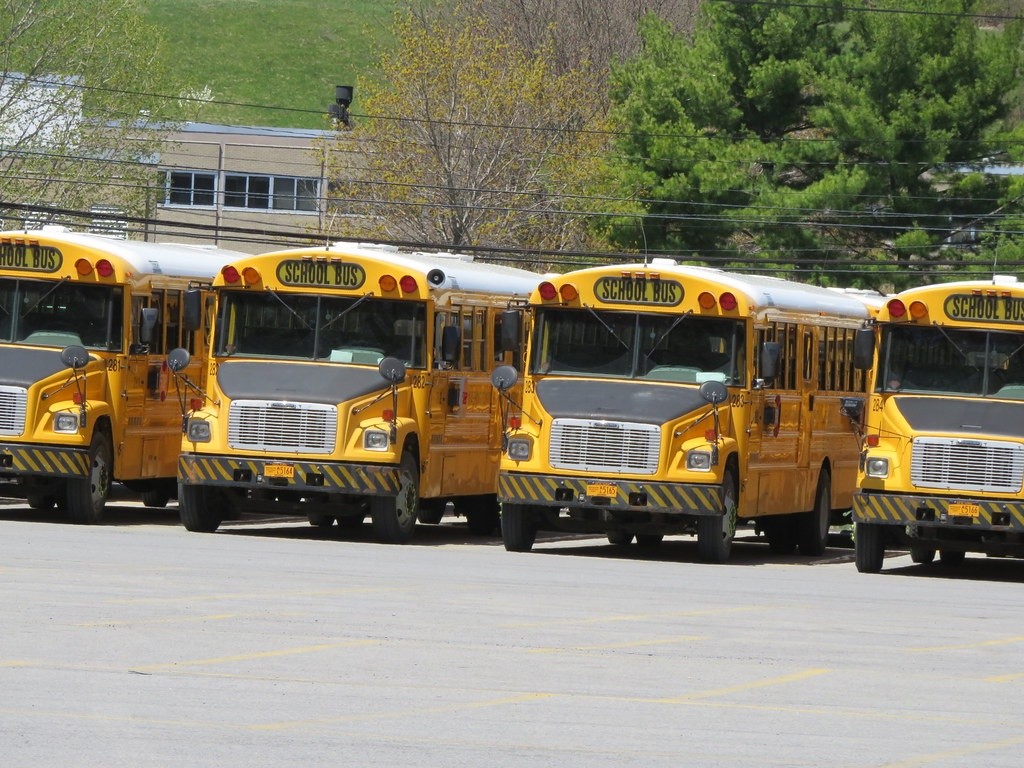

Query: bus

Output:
[165,241,562,544]
[490,258,899,562]
[838,275,1024,573]
[0,225,255,525]
